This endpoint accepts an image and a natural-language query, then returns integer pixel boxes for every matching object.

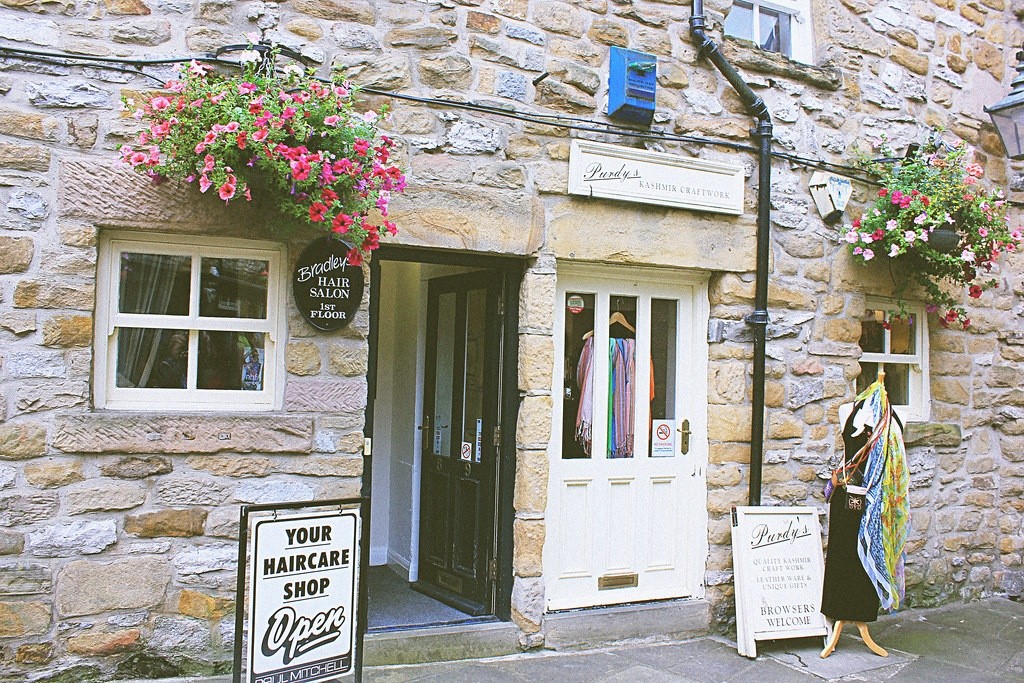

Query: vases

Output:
[929,230,959,253]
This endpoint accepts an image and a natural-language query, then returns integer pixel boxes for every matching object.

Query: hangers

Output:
[583,298,636,340]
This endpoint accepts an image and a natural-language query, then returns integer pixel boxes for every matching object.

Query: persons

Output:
[243,350,261,390]
[164,331,222,389]
[819,399,903,623]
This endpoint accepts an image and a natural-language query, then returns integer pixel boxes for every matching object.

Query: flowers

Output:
[114,34,406,267]
[832,125,1024,332]
